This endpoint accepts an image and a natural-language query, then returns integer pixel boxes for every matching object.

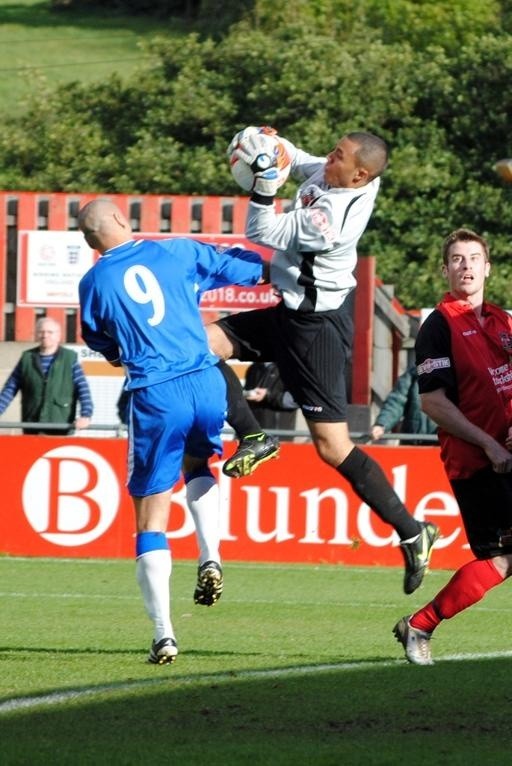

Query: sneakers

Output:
[392,614,436,666]
[148,638,178,666]
[193,560,224,608]
[222,431,280,477]
[398,521,441,595]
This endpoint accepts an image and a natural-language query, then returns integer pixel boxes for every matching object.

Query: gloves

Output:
[234,132,286,198]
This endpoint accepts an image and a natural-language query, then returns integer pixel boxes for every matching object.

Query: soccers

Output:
[230,128,290,195]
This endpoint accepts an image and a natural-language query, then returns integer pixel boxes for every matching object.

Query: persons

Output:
[236,359,298,443]
[74,198,272,664]
[370,366,442,447]
[390,229,512,666]
[1,315,95,436]
[204,126,442,595]
